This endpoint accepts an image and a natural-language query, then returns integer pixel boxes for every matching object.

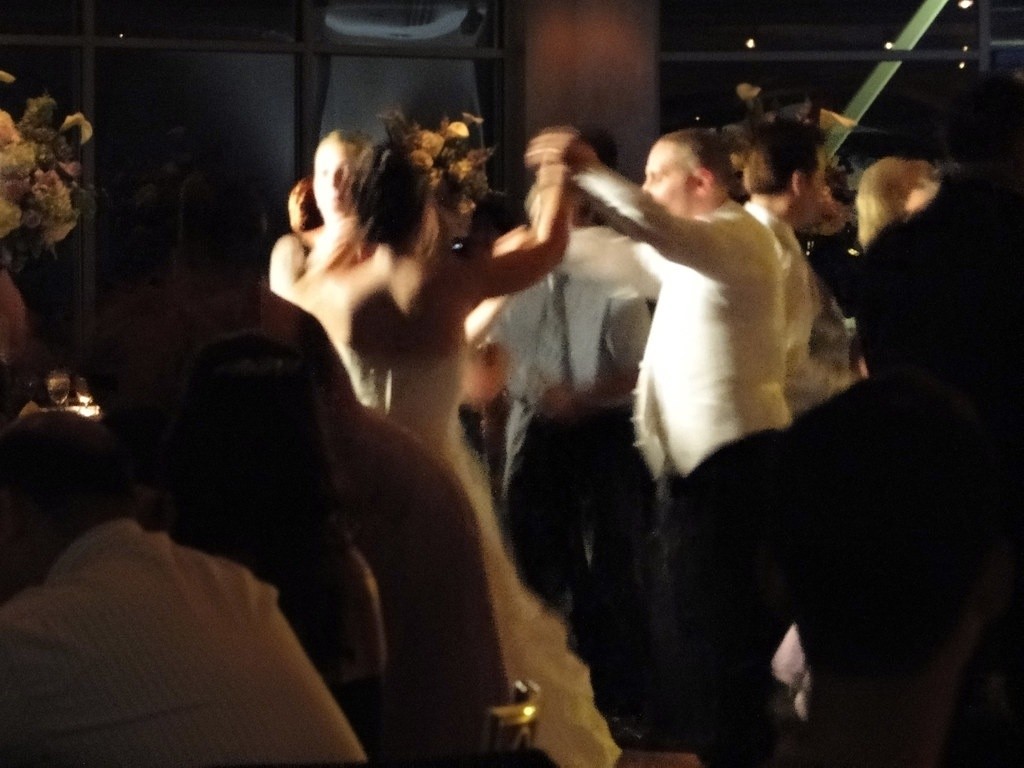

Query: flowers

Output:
[0,70,94,274]
[403,111,507,207]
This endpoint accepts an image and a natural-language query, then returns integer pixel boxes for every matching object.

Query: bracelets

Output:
[450,239,479,259]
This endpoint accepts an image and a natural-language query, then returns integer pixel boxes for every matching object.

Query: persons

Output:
[0,70,1024,768]
[0,412,368,768]
[524,127,795,768]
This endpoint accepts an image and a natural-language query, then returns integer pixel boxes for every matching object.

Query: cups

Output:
[75,376,94,416]
[47,372,69,410]
